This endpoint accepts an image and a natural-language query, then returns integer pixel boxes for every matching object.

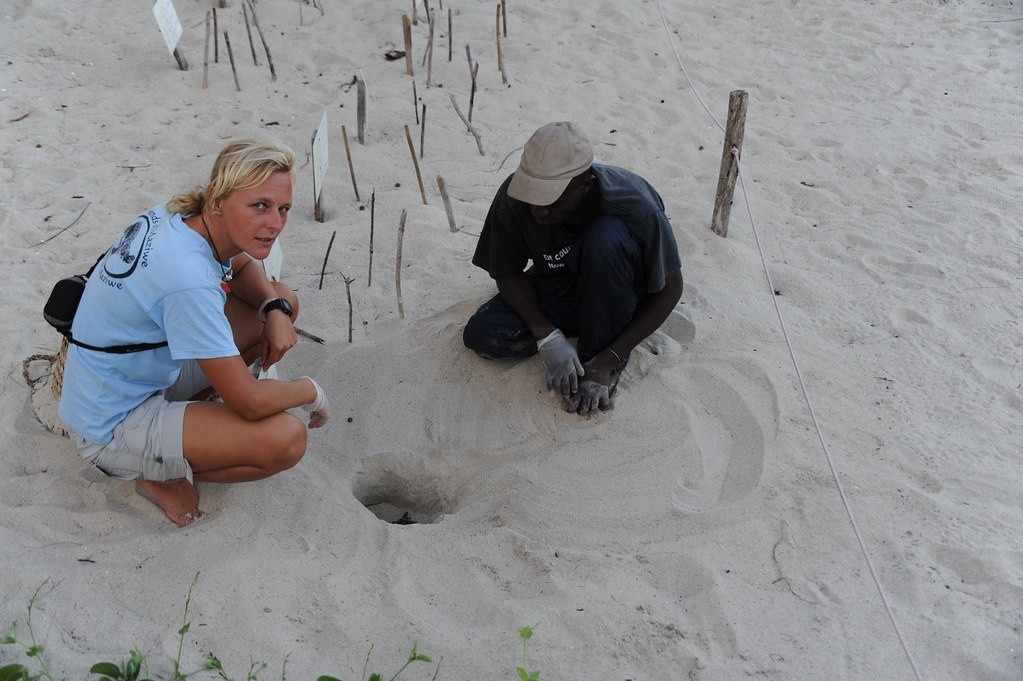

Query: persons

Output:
[59,140,332,529]
[463,122,683,416]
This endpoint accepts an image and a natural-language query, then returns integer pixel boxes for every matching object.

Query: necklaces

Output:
[202,214,233,282]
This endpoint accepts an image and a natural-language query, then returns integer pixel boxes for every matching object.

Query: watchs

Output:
[264,298,293,316]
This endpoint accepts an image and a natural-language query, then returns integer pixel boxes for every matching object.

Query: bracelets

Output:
[259,298,278,324]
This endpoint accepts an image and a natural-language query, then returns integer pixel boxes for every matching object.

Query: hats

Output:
[506,121,594,205]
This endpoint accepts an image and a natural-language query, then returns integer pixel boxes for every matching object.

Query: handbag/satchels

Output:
[42,275,88,332]
